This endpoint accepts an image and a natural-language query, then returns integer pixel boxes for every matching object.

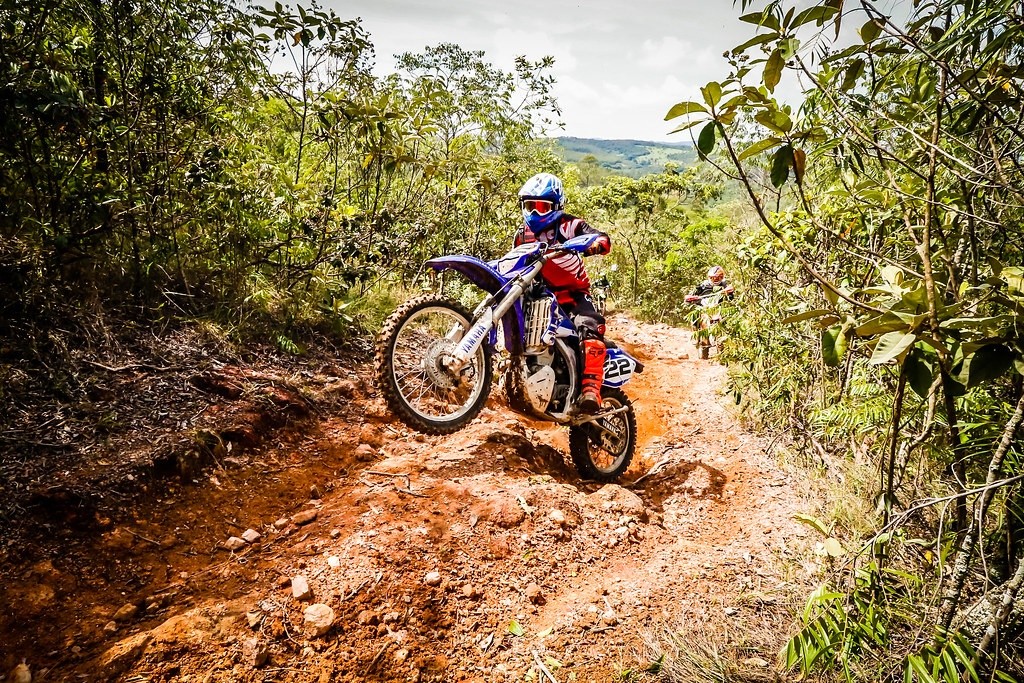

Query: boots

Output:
[577,339,606,413]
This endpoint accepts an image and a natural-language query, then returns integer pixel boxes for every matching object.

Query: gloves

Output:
[583,241,602,258]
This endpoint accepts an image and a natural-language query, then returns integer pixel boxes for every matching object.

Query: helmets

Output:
[708,266,724,285]
[518,173,565,233]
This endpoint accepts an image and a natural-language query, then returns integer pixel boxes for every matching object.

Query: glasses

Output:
[522,200,553,216]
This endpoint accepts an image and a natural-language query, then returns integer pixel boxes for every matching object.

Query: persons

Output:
[689,267,734,305]
[511,172,611,411]
[591,273,610,291]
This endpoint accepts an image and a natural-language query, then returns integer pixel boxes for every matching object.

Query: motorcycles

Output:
[371,244,639,486]
[684,294,735,361]
